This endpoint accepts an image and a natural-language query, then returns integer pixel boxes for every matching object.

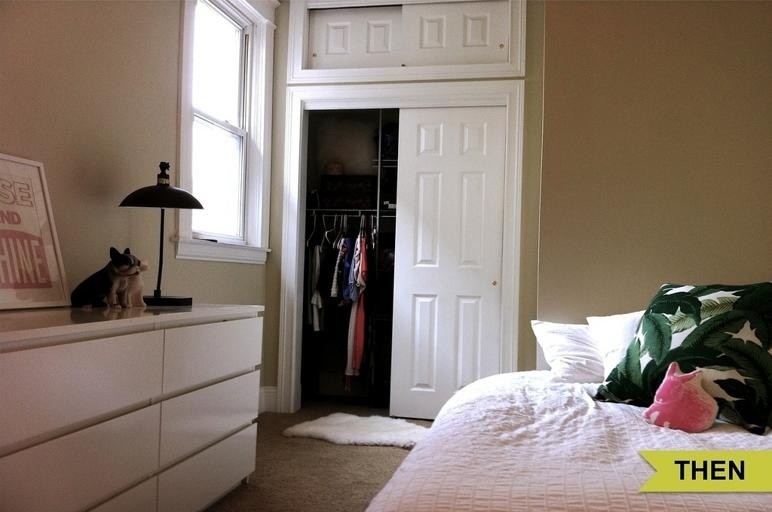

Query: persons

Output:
[643,360,719,433]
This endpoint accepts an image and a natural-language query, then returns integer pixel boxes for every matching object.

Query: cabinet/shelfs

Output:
[0,318,264,512]
[305,0,510,69]
[291,96,509,413]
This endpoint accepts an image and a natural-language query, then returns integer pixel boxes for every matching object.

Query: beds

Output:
[363,369,771,511]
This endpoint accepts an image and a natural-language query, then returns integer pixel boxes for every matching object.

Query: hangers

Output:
[306,215,365,248]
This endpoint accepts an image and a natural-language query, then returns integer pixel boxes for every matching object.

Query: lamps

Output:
[117,161,204,306]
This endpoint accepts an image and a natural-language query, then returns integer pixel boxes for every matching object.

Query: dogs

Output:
[123,262,149,308]
[70,247,141,309]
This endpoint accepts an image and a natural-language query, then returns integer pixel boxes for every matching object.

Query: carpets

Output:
[280,411,430,449]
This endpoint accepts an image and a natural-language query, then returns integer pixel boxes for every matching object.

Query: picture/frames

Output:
[0,152,71,311]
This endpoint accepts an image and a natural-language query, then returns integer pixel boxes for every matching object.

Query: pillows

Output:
[529,281,772,436]
